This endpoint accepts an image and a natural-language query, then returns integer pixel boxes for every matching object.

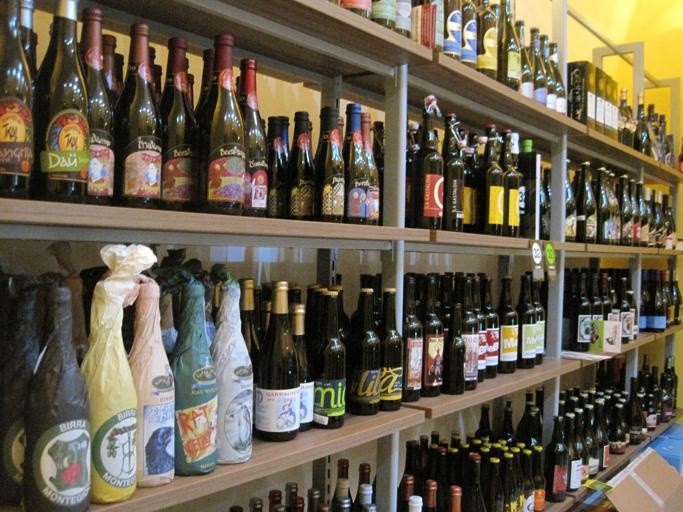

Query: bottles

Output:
[0,244,681,511]
[0,0,680,252]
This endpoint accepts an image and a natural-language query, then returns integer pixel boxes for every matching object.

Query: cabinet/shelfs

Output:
[0,0,683,512]
